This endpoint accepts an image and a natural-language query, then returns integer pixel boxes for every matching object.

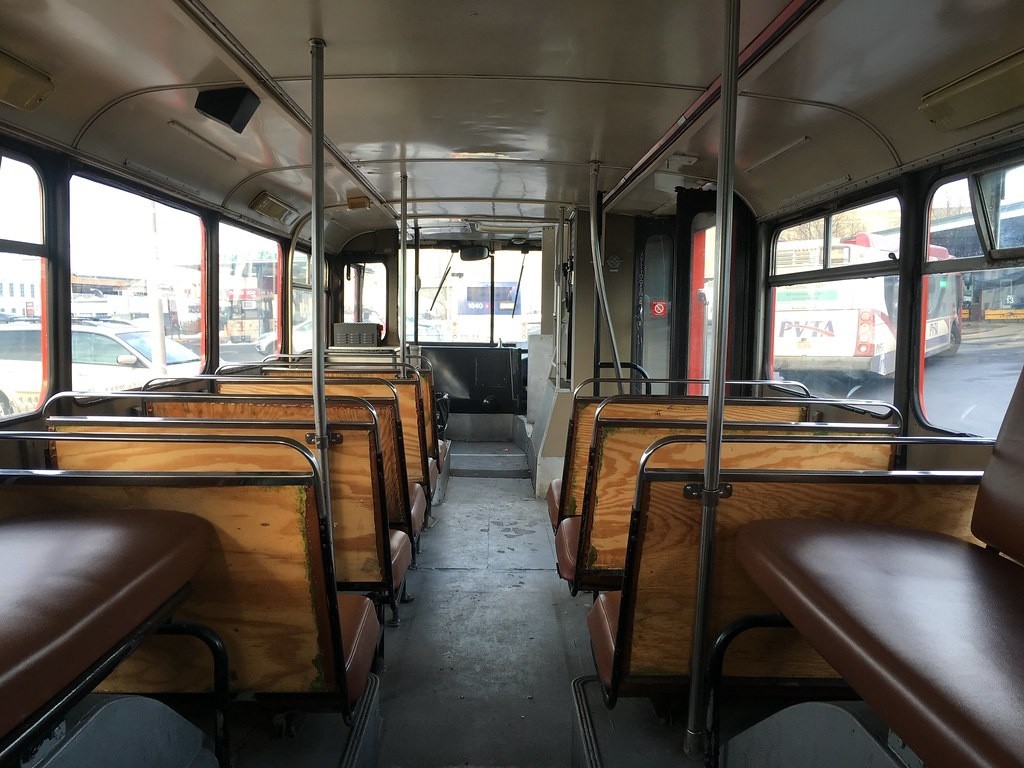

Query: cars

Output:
[255,315,386,357]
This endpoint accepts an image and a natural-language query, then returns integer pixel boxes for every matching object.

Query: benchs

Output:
[0,347,453,768]
[547,378,1024,768]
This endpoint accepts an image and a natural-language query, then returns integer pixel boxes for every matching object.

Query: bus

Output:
[772,232,961,385]
[419,269,528,344]
[224,288,278,343]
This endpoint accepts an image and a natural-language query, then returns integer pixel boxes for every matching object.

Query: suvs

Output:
[0,315,201,418]
